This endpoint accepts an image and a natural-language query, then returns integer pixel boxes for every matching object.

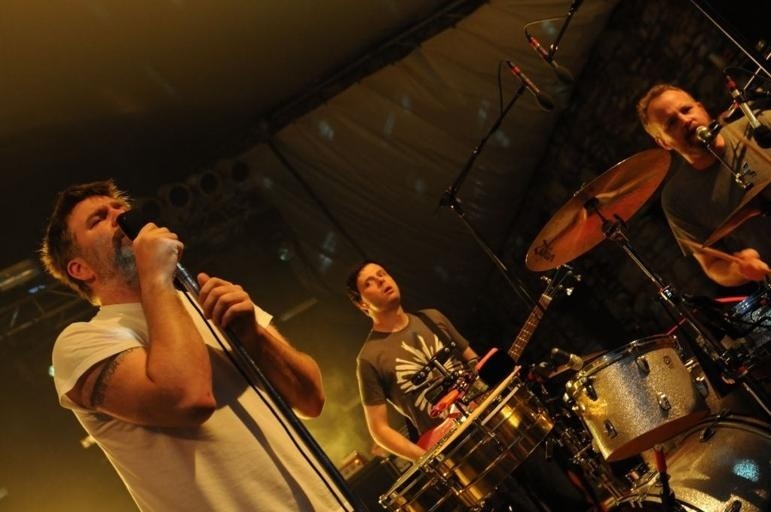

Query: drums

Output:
[565,333,713,462]
[620,411,770,507]
[429,374,557,463]
[379,458,455,507]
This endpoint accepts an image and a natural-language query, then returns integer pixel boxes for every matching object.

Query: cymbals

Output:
[703,178,771,244]
[526,149,673,272]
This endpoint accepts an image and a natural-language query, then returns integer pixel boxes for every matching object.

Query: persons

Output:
[634,82,770,287]
[41,178,365,512]
[342,259,592,512]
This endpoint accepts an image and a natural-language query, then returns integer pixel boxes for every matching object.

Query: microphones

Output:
[727,77,771,149]
[551,347,584,371]
[116,208,190,282]
[529,37,577,88]
[695,125,711,144]
[505,58,556,110]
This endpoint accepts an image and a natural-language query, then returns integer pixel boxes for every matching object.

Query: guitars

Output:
[414,262,585,452]
